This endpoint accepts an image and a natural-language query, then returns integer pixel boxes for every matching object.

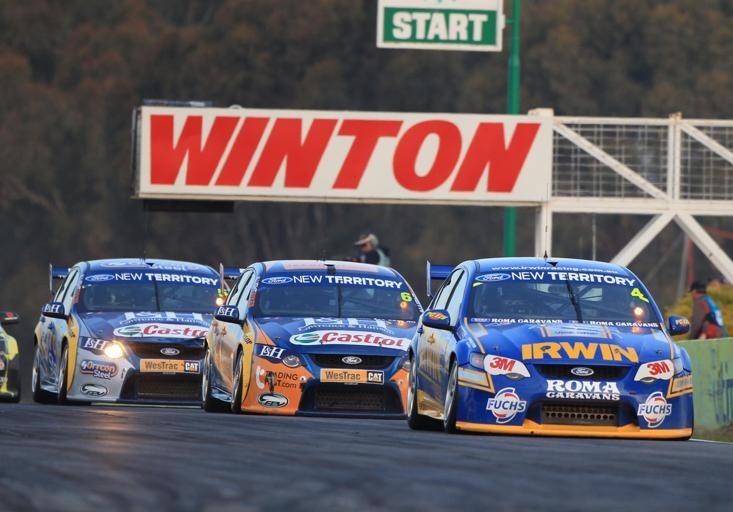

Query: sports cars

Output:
[407,256,693,440]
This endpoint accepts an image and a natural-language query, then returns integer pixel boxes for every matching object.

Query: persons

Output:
[349,232,390,267]
[684,278,728,342]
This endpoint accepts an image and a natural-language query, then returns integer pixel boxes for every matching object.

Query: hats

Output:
[354,232,380,248]
[687,282,706,293]
